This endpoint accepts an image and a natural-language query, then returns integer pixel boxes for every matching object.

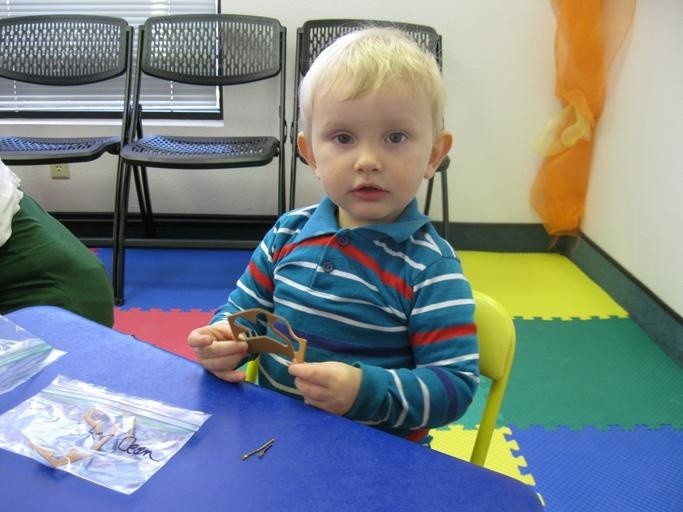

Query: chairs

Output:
[446,287,519,468]
[290,18,451,243]
[0,13,148,305]
[116,14,287,303]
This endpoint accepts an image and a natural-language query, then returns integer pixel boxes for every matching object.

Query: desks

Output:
[0,301,547,512]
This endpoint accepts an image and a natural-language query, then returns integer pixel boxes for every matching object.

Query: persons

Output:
[0,162,115,328]
[186,24,479,447]
